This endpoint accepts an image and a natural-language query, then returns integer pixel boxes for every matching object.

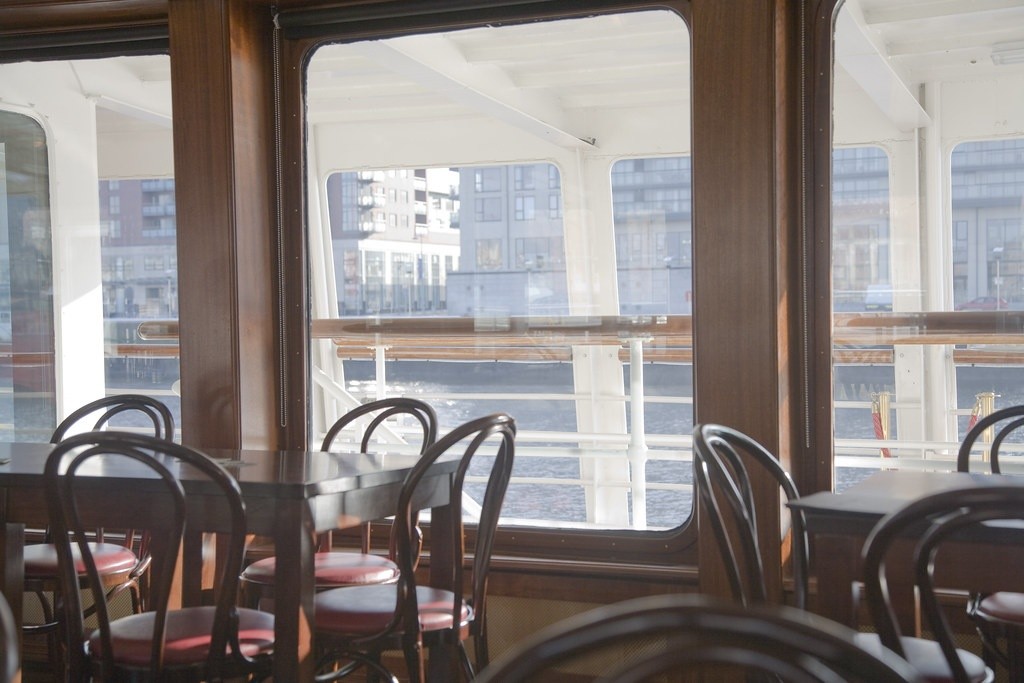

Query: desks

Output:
[785,470,1024,624]
[0,441,470,683]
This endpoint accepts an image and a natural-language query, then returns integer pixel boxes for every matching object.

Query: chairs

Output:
[0,389,1024,683]
